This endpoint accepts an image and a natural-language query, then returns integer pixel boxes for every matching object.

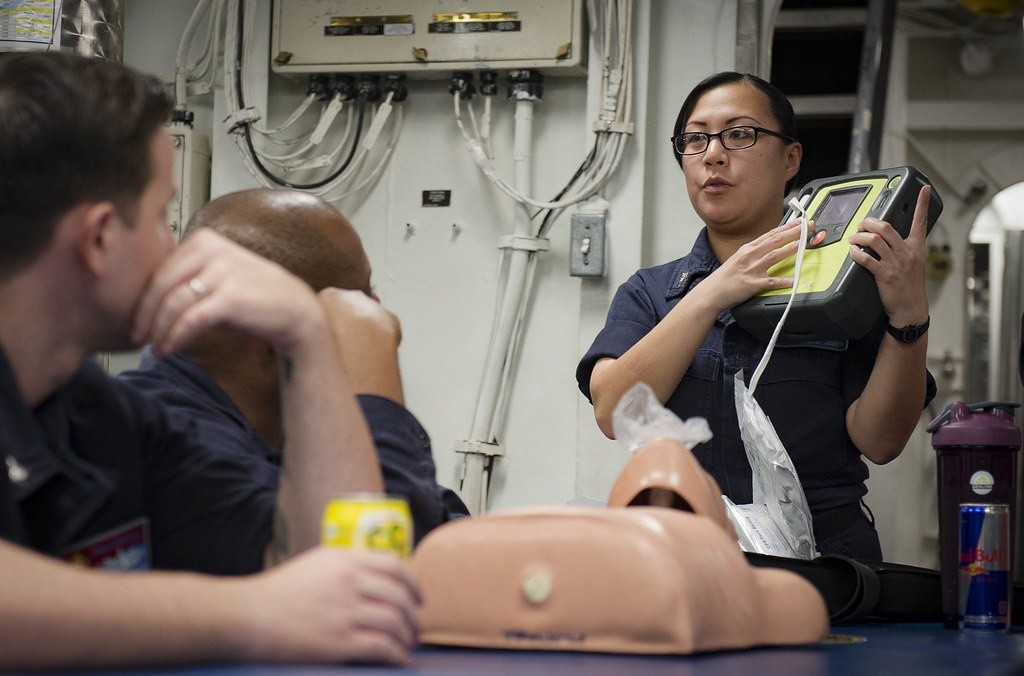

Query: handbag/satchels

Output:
[618,369,817,558]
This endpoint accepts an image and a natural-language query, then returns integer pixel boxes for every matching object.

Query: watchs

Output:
[882,315,928,347]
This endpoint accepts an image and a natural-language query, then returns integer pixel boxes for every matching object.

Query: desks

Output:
[49,624,1024,676]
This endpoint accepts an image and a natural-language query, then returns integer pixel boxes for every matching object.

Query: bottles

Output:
[926,401,1024,627]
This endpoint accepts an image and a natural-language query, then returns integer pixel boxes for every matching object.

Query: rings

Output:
[187,277,207,301]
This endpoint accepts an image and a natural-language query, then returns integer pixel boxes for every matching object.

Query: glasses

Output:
[671,126,795,155]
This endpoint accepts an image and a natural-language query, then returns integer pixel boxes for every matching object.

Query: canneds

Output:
[957,502,1010,634]
[319,493,414,564]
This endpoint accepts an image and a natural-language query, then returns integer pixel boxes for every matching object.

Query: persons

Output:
[0,51,428,665]
[116,187,473,554]
[575,70,934,571]
